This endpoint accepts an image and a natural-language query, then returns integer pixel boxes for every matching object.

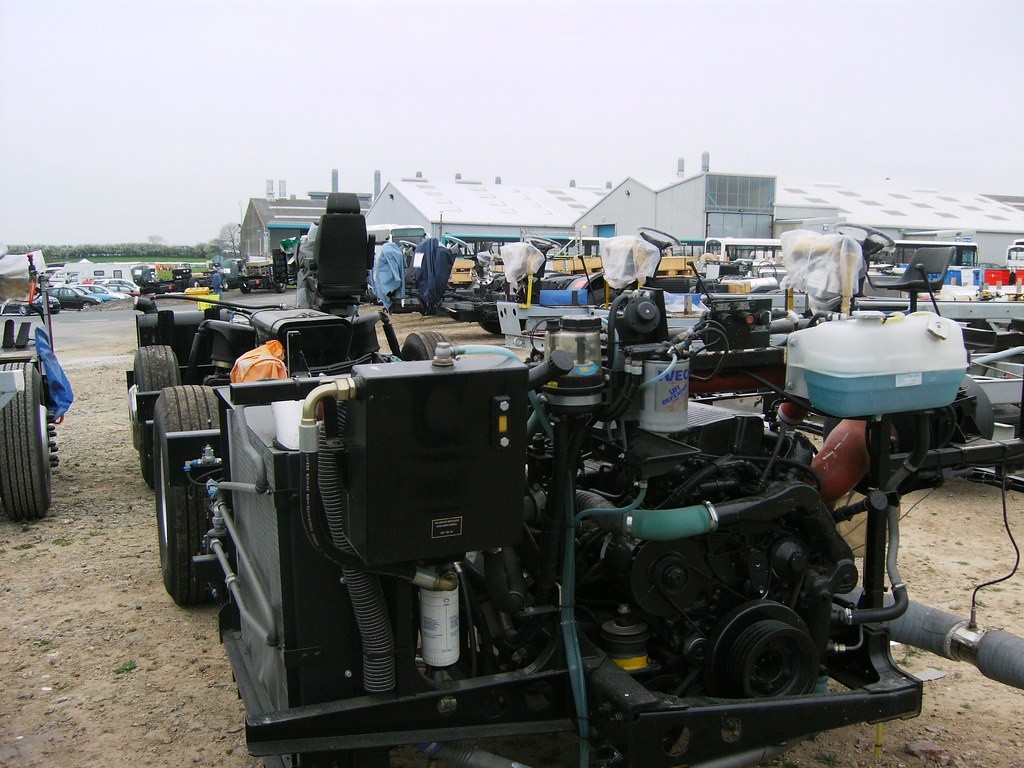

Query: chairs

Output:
[695,264,739,291]
[404,238,439,290]
[874,247,957,313]
[304,193,376,315]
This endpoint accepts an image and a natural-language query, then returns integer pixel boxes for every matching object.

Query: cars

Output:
[40,277,141,310]
[0,292,61,316]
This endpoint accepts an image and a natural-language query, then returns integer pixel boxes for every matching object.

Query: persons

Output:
[213,269,224,301]
